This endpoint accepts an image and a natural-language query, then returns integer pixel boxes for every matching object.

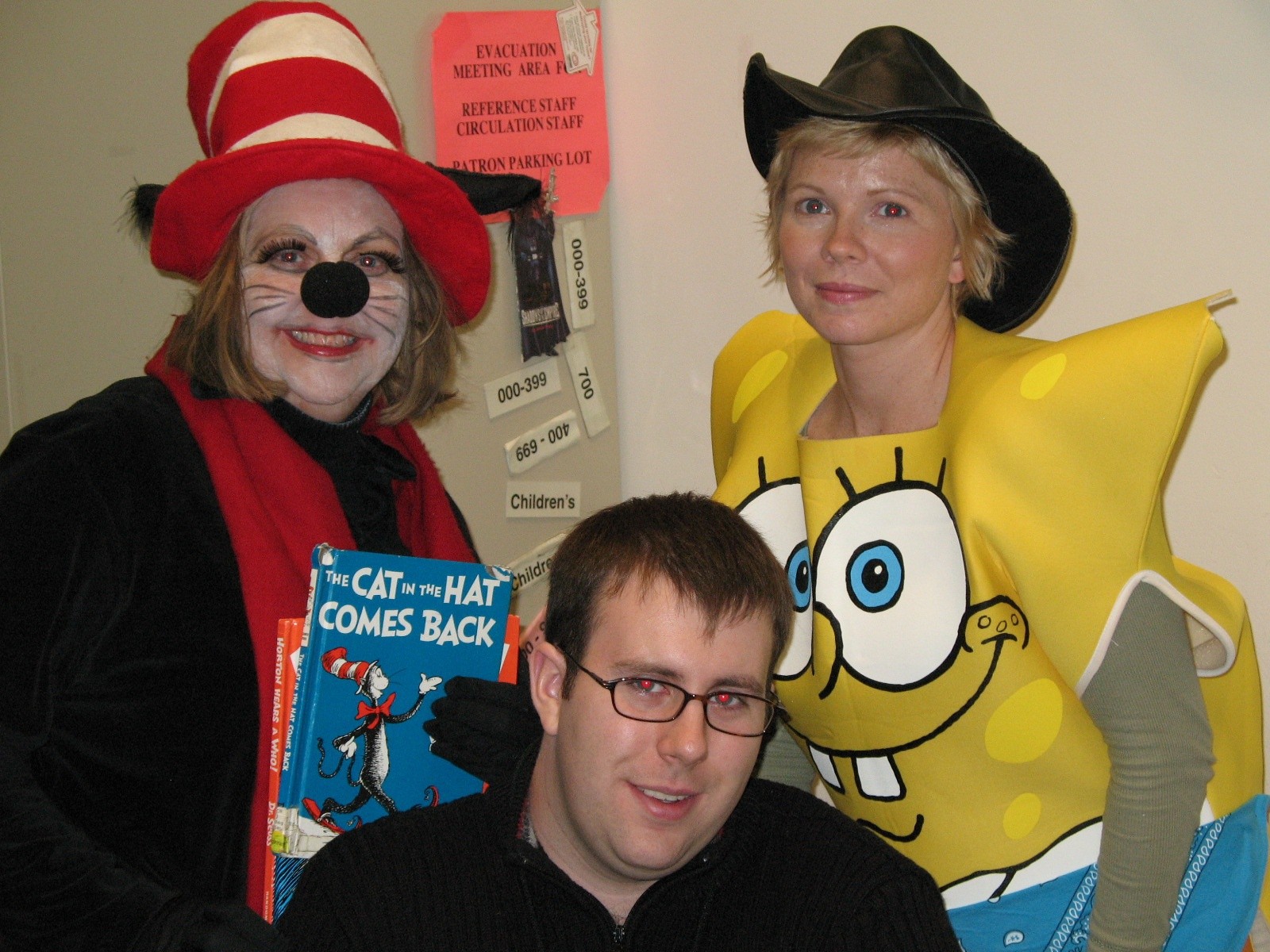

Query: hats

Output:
[151,0,494,329]
[742,25,1074,332]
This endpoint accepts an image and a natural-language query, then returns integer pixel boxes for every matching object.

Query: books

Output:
[247,542,522,923]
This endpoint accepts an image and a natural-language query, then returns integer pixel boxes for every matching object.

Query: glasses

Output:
[557,644,779,737]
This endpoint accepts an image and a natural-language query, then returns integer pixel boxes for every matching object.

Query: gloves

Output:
[425,653,541,783]
[175,893,294,952]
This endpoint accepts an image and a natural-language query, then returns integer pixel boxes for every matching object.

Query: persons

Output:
[709,26,1270,952]
[276,491,961,952]
[0,0,539,952]
